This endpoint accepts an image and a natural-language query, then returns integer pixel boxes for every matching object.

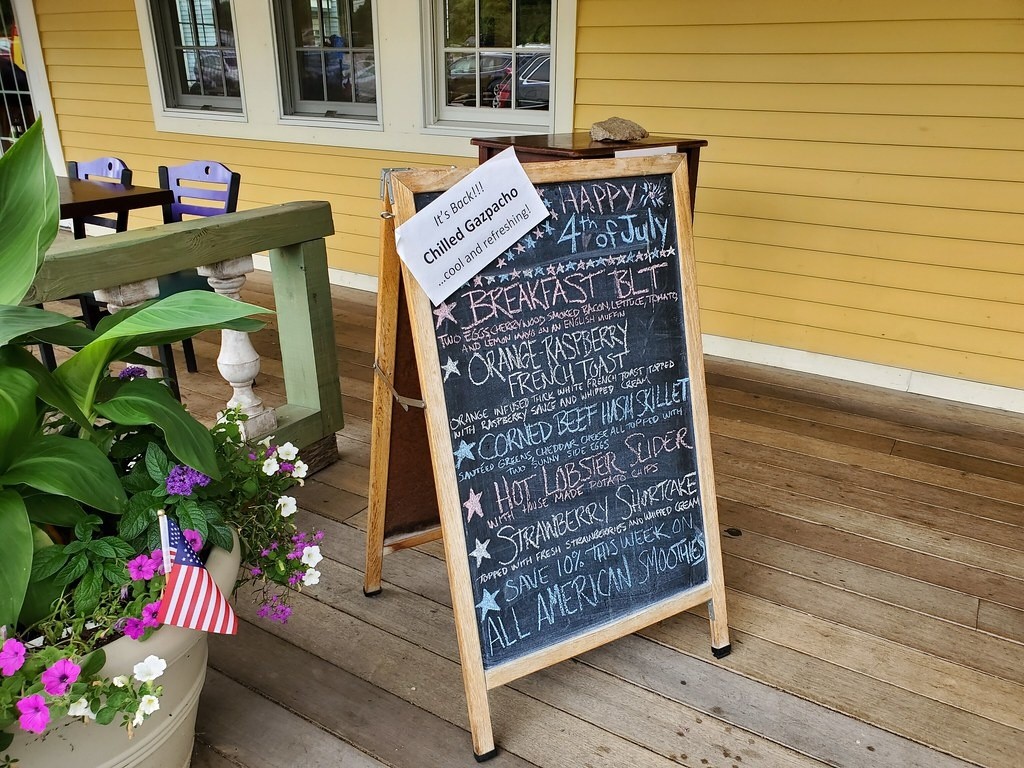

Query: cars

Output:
[299,49,375,101]
[194,48,240,88]
[445,50,549,108]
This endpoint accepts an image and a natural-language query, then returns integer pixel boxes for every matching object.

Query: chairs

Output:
[54,157,240,404]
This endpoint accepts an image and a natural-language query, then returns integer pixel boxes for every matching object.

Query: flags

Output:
[153,519,237,635]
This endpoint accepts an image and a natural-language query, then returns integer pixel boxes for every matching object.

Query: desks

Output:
[29,176,197,374]
[471,132,708,226]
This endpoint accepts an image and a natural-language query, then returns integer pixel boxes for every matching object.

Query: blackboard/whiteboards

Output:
[367,152,724,692]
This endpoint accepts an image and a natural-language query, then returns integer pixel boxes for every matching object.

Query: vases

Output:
[0,521,242,768]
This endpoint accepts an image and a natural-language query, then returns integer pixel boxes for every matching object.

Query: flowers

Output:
[0,112,322,768]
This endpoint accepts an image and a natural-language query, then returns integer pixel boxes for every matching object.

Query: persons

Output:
[341,4,376,102]
